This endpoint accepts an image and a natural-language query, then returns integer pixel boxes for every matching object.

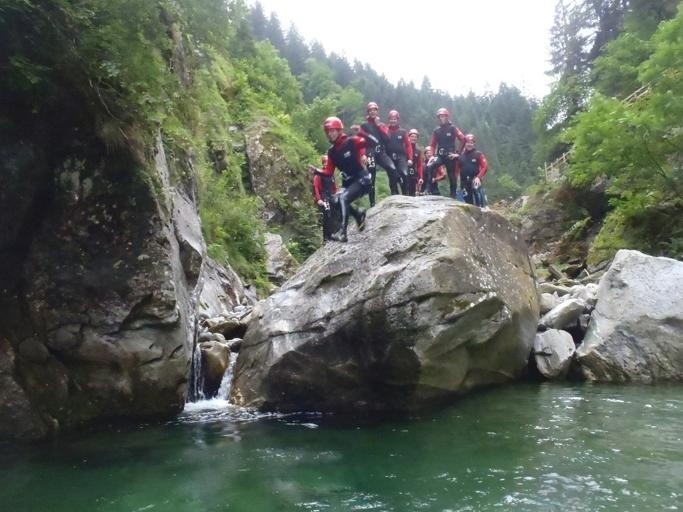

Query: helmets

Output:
[367,101,378,110]
[464,134,475,143]
[436,107,447,117]
[322,116,343,130]
[387,110,399,119]
[408,129,418,138]
[423,145,432,154]
[321,155,329,164]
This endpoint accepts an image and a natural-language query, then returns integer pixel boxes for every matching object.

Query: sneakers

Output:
[330,230,347,242]
[356,206,366,232]
[418,189,432,196]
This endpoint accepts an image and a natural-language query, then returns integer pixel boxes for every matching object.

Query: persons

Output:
[305,100,488,243]
